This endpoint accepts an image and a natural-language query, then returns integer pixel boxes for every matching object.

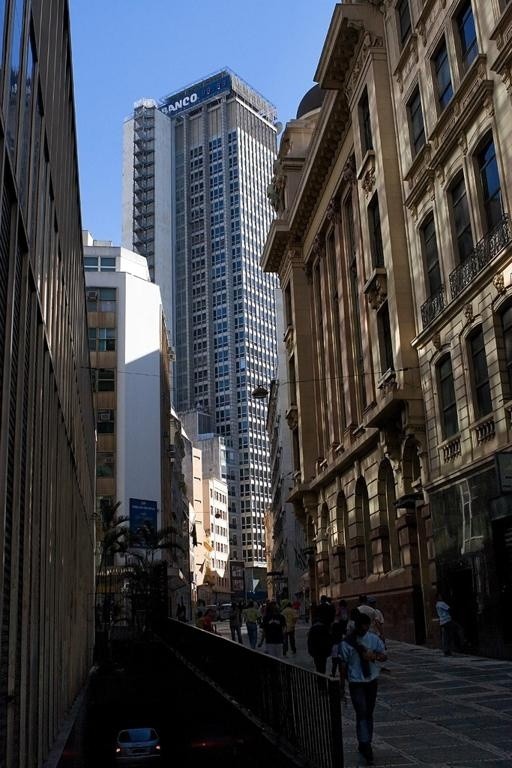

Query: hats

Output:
[197,598,277,622]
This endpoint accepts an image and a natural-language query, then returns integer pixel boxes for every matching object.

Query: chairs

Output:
[358,743,375,761]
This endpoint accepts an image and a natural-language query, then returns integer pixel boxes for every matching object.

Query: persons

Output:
[177,596,298,659]
[337,612,388,762]
[307,595,386,676]
[431,596,452,655]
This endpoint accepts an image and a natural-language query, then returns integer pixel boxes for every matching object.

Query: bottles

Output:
[354,593,368,601]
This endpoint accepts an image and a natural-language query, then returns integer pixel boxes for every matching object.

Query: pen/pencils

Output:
[113,727,162,767]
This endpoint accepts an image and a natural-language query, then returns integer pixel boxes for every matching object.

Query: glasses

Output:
[252,383,267,401]
